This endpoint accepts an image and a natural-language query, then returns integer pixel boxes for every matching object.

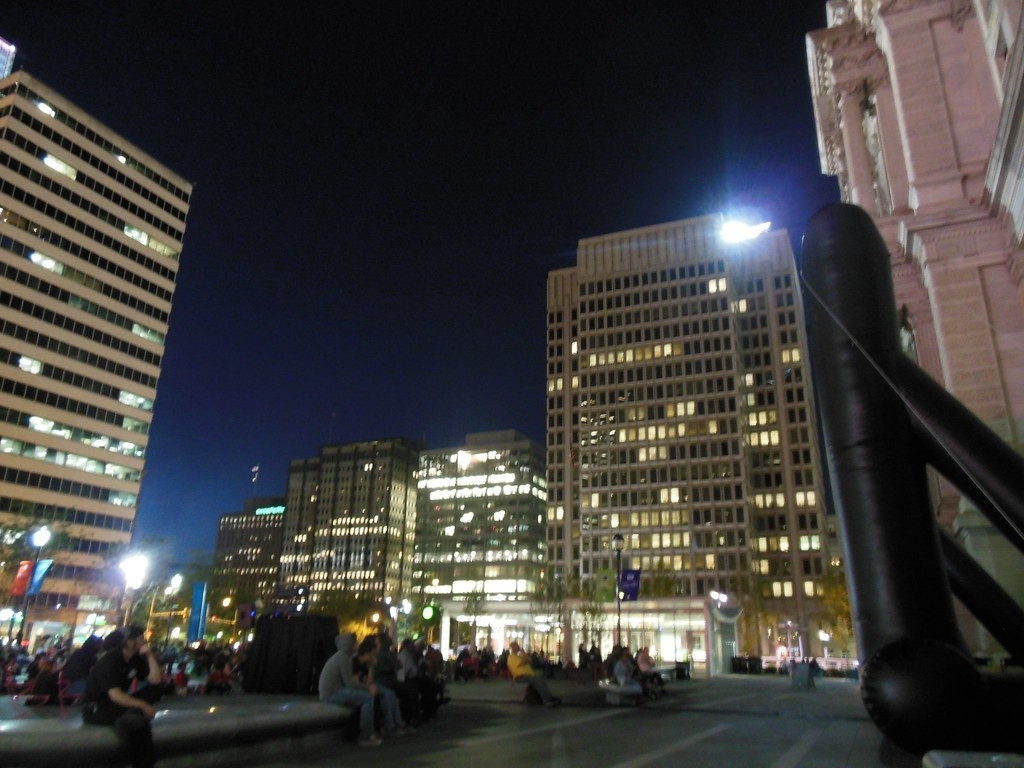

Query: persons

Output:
[0,635,236,707]
[319,632,449,746]
[506,643,563,707]
[496,641,576,679]
[125,621,161,706]
[82,628,156,768]
[577,639,668,700]
[454,645,494,683]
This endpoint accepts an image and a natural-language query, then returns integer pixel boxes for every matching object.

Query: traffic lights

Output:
[422,605,439,627]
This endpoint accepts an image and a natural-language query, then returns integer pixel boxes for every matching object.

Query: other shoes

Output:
[358,733,382,747]
[386,726,409,737]
[546,697,561,705]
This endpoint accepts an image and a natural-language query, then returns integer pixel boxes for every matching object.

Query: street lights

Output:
[611,532,625,647]
[13,523,52,648]
